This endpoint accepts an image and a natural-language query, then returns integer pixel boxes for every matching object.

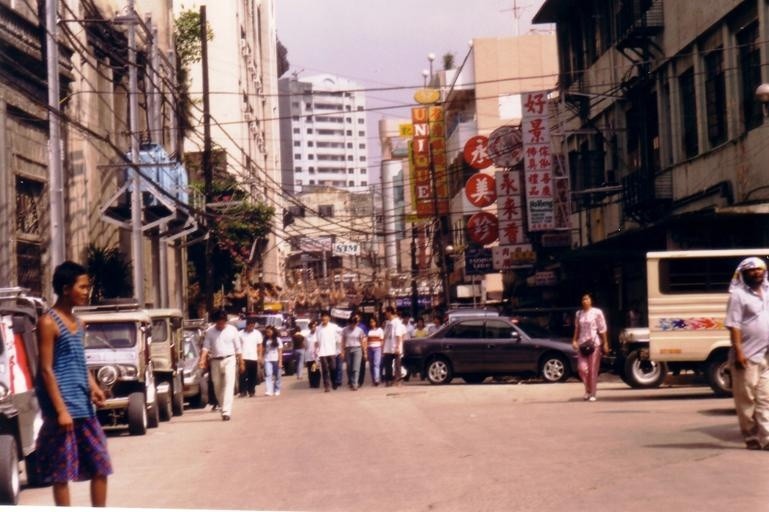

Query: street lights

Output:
[45,14,144,291]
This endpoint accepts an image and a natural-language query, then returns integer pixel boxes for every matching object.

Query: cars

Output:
[178,309,332,405]
[402,315,606,382]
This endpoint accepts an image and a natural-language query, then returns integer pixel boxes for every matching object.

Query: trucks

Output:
[646,248,769,397]
[444,308,498,327]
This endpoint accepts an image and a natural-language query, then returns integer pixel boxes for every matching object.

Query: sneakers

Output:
[309,379,403,392]
[221,413,230,422]
[238,389,281,398]
[583,391,597,403]
[746,440,762,450]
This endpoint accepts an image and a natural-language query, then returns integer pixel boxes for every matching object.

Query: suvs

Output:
[612,325,704,386]
[0,288,186,501]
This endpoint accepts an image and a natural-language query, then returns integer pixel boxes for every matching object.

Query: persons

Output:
[199,308,244,422]
[235,318,264,398]
[33,260,115,506]
[263,326,284,396]
[724,255,769,451]
[289,308,448,393]
[205,358,219,411]
[571,293,609,401]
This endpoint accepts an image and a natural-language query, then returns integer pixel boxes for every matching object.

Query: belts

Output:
[212,355,233,360]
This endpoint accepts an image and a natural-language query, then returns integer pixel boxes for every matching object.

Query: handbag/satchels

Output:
[578,339,595,355]
